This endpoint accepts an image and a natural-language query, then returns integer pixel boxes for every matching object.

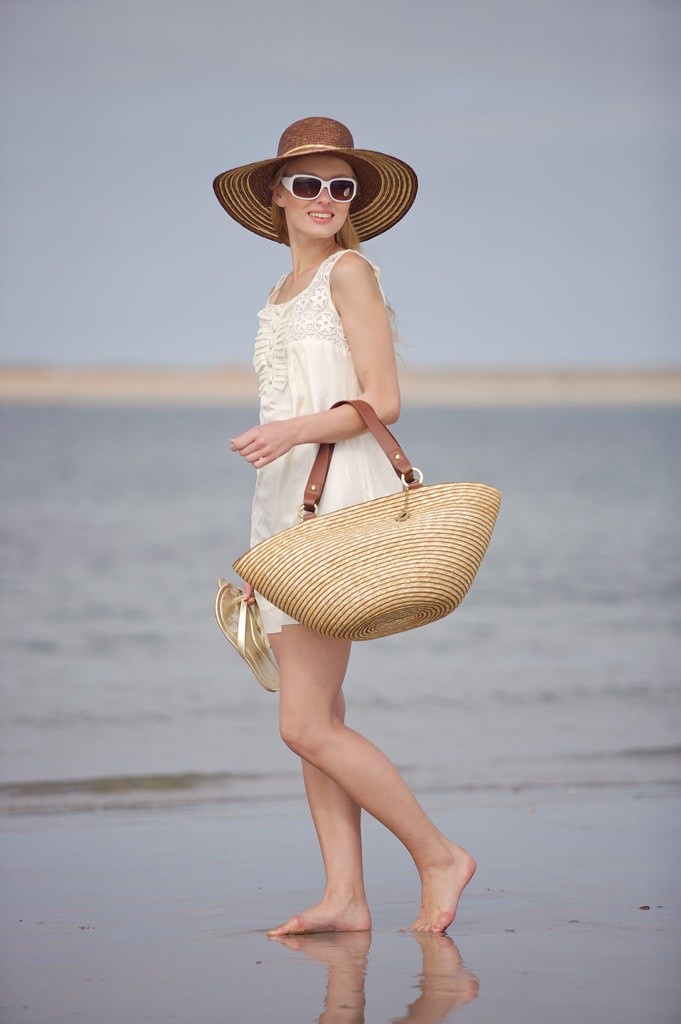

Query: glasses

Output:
[277,175,358,203]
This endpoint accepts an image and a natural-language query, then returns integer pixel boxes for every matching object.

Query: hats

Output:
[212,116,418,246]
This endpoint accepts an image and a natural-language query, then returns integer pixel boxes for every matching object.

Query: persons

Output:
[212,118,474,934]
[269,936,481,1024]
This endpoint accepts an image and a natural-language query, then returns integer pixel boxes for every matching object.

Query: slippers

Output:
[213,580,285,693]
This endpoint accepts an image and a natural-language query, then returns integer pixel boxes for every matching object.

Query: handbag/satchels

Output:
[230,402,507,642]
